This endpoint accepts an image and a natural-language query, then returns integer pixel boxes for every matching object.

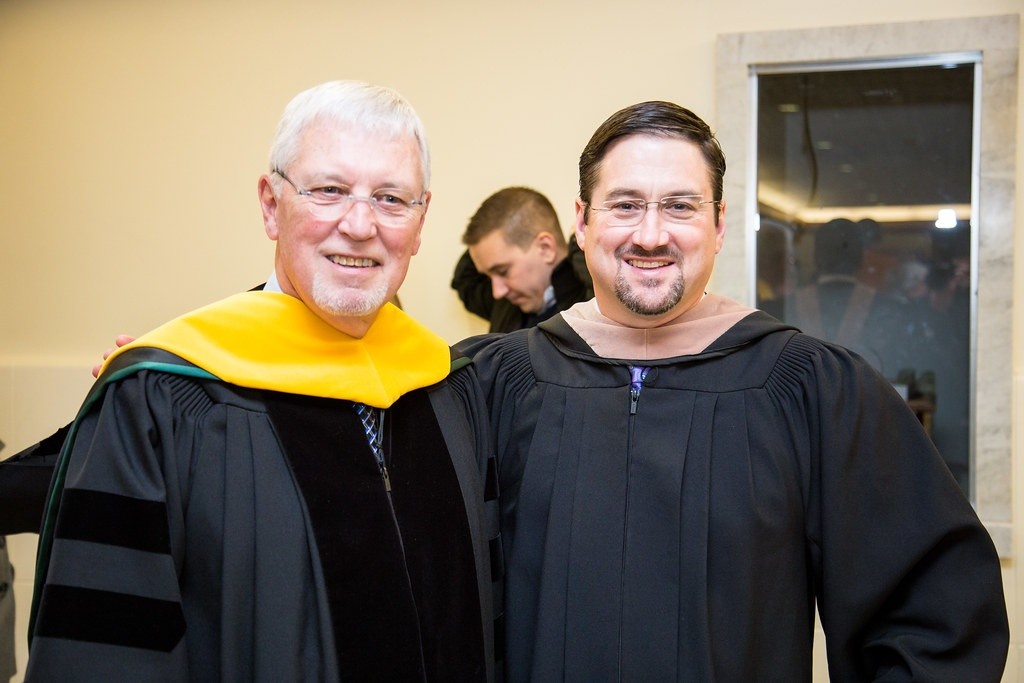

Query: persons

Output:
[472,101,1011,683]
[23,80,505,683]
[451,186,596,334]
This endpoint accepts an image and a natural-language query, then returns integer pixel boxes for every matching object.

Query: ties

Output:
[355,400,384,466]
[629,367,648,395]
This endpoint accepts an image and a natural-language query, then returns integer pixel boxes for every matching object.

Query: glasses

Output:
[586,195,721,226]
[272,168,423,227]
